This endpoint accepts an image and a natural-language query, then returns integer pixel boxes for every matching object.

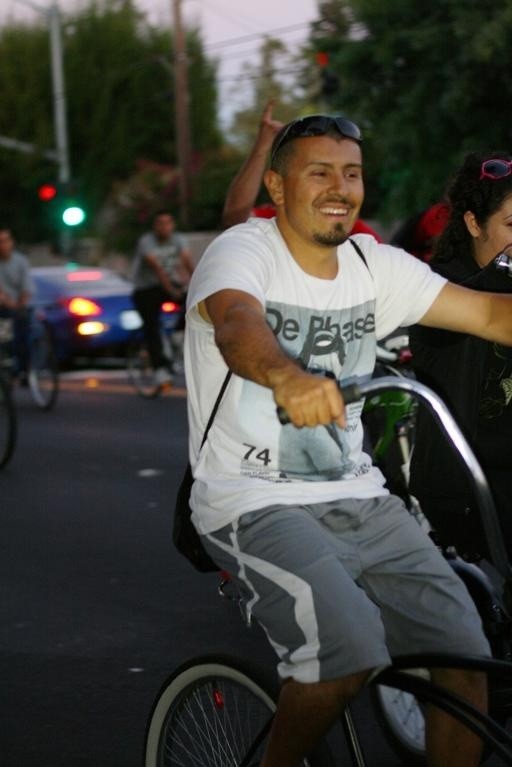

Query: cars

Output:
[24,262,182,372]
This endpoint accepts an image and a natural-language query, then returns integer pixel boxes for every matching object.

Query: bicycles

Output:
[141,373,510,766]
[126,283,195,401]
[1,302,61,413]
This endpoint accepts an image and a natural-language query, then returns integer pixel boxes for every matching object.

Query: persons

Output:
[130,212,196,391]
[390,161,467,267]
[404,152,512,566]
[1,225,40,375]
[185,114,511,766]
[223,99,385,246]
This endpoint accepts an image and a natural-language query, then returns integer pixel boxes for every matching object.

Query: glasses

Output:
[478,158,512,181]
[268,116,364,169]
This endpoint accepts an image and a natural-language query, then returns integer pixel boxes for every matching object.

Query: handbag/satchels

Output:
[171,461,224,574]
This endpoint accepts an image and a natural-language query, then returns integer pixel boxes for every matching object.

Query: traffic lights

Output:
[27,179,90,234]
